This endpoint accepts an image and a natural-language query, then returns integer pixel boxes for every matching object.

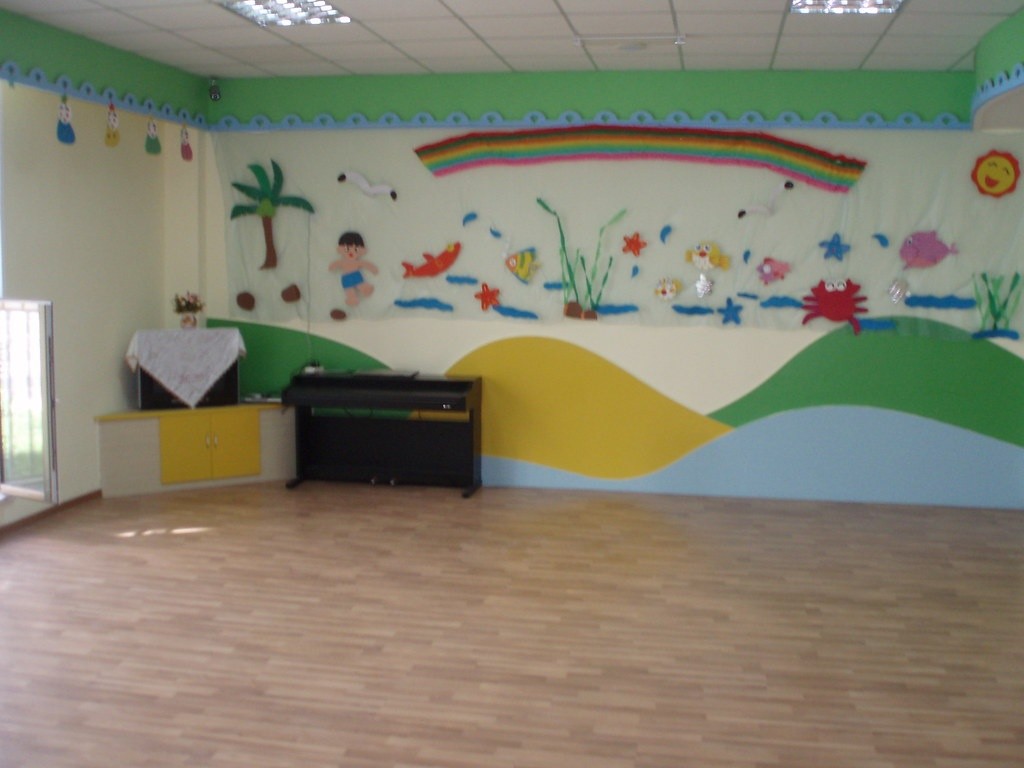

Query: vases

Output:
[179,312,197,329]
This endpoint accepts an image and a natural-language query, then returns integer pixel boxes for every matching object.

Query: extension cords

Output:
[304,365,323,374]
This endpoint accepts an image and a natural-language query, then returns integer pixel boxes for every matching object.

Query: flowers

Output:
[173,291,205,314]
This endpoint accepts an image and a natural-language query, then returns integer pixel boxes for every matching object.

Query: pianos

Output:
[280,371,485,499]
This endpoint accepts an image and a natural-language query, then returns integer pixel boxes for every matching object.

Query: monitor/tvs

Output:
[136,327,239,410]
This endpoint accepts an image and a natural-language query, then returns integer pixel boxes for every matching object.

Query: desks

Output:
[93,404,296,500]
